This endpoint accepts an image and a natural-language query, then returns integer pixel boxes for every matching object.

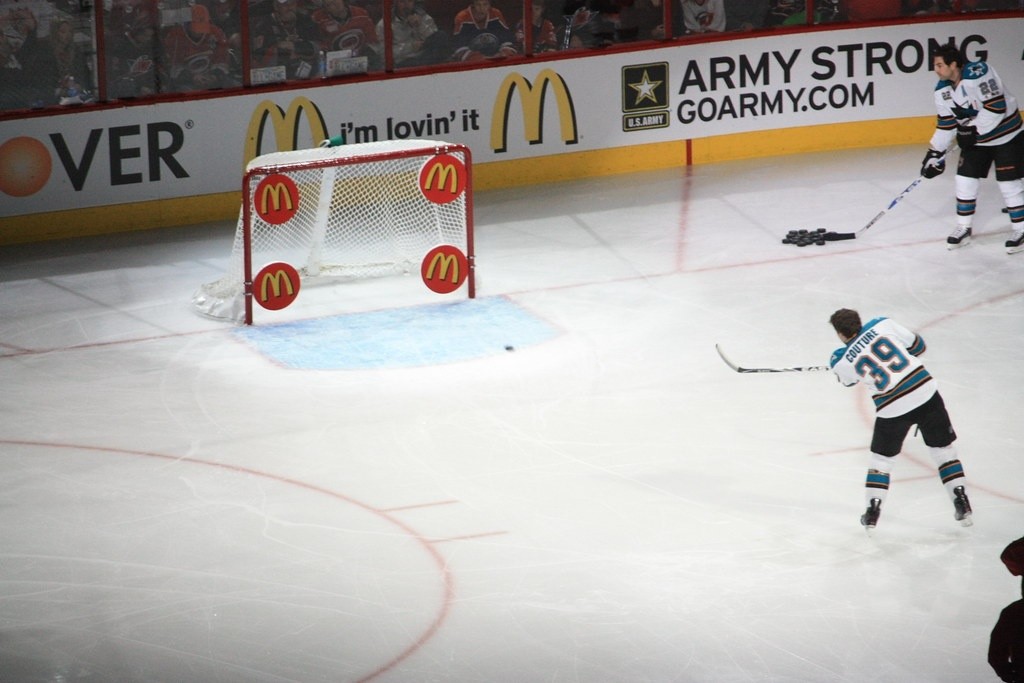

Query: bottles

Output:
[317,50,325,77]
[831,1,843,22]
[67,77,77,98]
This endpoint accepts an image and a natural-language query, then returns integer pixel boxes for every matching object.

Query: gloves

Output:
[920,148,946,178]
[956,124,976,152]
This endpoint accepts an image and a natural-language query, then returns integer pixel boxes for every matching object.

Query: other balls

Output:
[781,227,837,248]
[505,344,514,352]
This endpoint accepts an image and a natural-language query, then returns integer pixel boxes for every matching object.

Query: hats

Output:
[191,5,211,34]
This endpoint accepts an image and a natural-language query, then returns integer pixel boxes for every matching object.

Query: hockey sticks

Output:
[714,342,830,375]
[821,143,962,242]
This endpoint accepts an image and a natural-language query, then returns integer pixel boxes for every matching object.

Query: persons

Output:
[830,309,970,529]
[988,576,1024,683]
[1,0,990,114]
[921,45,1024,254]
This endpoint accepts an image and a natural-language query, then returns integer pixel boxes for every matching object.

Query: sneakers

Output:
[948,227,972,249]
[953,485,973,526]
[1006,229,1024,254]
[861,498,882,536]
